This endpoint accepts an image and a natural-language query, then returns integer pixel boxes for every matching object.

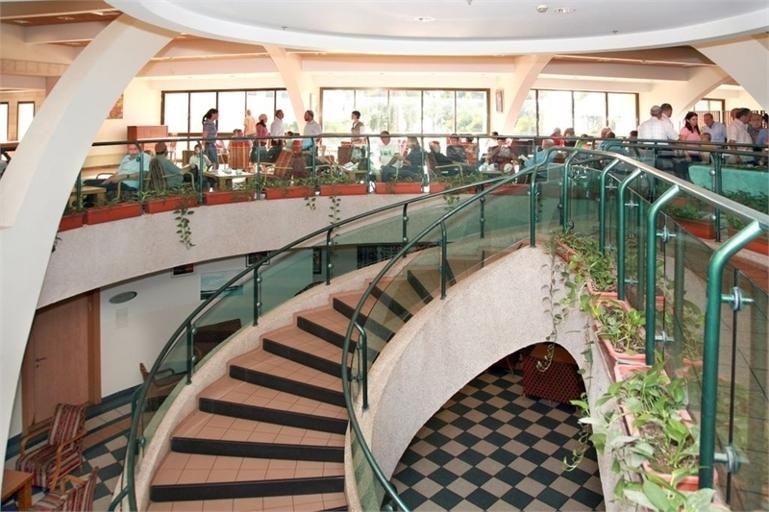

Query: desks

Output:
[0,470,33,512]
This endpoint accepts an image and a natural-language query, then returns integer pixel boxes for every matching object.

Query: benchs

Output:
[193,318,242,360]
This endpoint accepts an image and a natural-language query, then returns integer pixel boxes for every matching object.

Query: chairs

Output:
[138,361,188,408]
[32,467,98,512]
[16,403,85,493]
[79,126,619,205]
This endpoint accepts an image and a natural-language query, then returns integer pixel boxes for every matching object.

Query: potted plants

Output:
[582,265,619,296]
[626,279,667,317]
[550,233,600,274]
[58,182,200,251]
[597,359,702,492]
[655,303,704,382]
[589,295,647,369]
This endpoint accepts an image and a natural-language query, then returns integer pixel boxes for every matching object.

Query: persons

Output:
[83,103,769,207]
